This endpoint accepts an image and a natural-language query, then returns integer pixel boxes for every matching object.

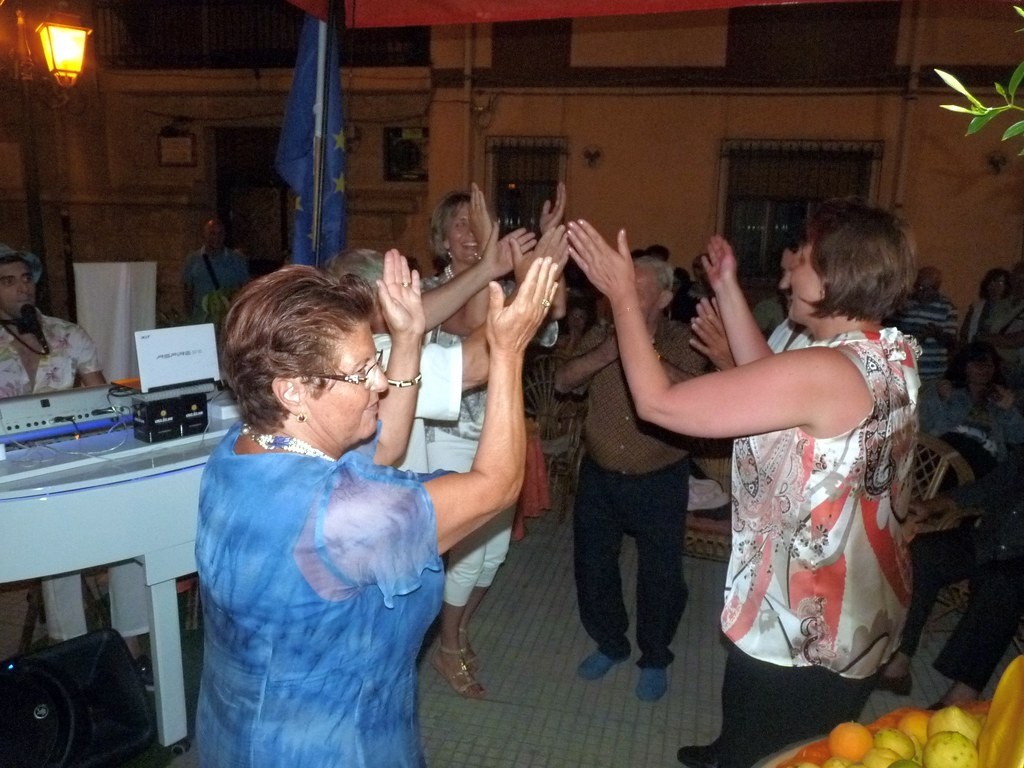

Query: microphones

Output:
[21,304,50,353]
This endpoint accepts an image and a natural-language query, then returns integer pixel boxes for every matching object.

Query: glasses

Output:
[303,349,383,384]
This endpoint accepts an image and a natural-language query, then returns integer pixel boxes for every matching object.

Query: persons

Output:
[1,240,160,696]
[313,177,790,703]
[564,196,922,767]
[876,257,1023,712]
[190,246,561,768]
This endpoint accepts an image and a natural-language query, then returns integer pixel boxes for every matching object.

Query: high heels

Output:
[429,643,486,697]
[456,639,480,673]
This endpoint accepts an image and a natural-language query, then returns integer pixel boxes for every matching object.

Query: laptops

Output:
[111,323,220,394]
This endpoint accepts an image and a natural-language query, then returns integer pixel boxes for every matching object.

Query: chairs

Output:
[523,353,588,524]
[921,506,1024,655]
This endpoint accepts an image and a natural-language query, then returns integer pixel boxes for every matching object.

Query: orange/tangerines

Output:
[897,710,929,745]
[829,719,872,763]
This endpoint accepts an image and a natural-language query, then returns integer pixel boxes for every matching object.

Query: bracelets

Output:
[382,370,422,387]
[615,304,641,317]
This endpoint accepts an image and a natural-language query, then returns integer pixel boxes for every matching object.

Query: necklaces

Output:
[444,266,451,280]
[240,420,340,463]
[447,264,454,278]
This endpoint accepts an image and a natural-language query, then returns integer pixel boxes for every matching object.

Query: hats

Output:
[0,242,43,283]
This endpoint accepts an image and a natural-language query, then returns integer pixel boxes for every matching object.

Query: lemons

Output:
[792,704,981,768]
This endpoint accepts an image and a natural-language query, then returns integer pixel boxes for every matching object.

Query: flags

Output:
[274,13,348,271]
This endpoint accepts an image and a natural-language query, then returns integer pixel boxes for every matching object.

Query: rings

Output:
[402,282,412,288]
[541,299,551,307]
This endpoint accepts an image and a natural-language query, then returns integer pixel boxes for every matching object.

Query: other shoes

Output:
[875,668,913,696]
[577,651,631,679]
[678,745,718,768]
[637,667,667,702]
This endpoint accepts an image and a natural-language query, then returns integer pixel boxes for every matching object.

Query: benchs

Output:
[682,430,977,563]
